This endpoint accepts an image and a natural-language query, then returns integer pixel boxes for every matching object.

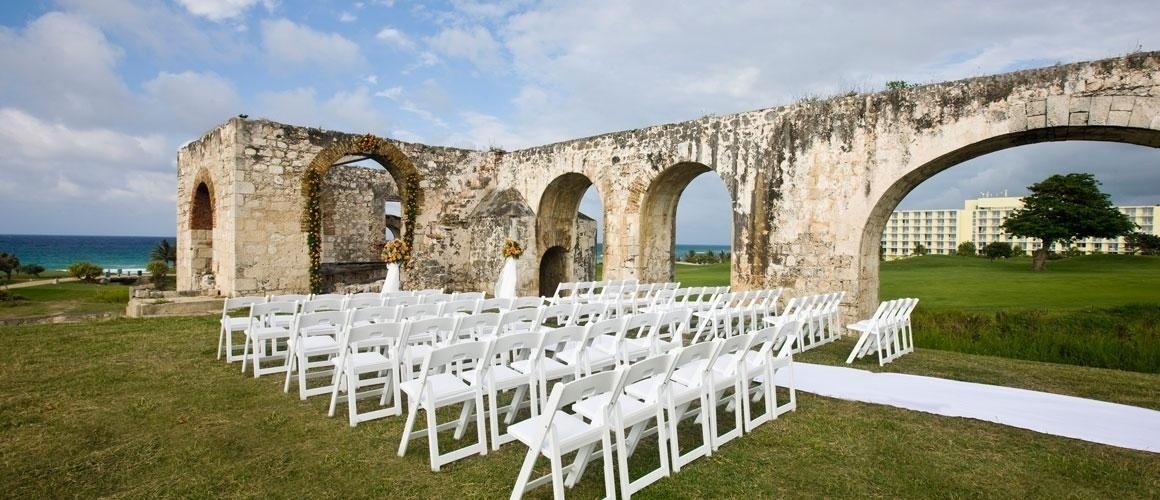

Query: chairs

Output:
[216,278,920,500]
